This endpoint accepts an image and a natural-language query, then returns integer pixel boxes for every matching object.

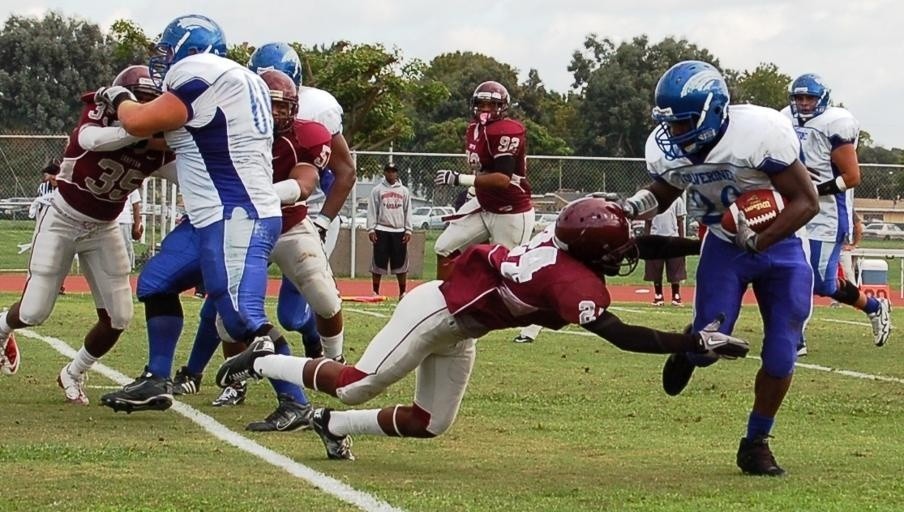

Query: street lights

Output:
[603,167,606,191]
[889,171,894,200]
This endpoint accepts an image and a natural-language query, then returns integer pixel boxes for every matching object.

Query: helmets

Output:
[111,15,230,100]
[469,80,512,124]
[554,198,640,278]
[246,42,303,136]
[787,73,832,120]
[650,59,731,161]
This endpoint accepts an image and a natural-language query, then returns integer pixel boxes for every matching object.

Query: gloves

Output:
[588,192,635,216]
[94,87,137,113]
[734,211,759,251]
[435,169,459,186]
[691,311,748,362]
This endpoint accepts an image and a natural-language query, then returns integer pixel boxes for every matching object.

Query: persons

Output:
[587,59,820,475]
[780,73,892,357]
[642,194,687,307]
[434,80,542,343]
[457,190,489,246]
[217,196,749,461]
[830,208,863,308]
[369,162,414,301]
[4,14,357,434]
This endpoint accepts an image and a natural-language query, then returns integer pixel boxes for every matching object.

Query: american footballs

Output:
[720,189,790,235]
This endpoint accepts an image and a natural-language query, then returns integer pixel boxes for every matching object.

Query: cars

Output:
[411,207,456,230]
[346,211,368,230]
[532,190,645,236]
[861,224,904,241]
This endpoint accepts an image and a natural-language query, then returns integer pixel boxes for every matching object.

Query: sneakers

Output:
[868,298,894,347]
[58,360,90,404]
[176,335,276,407]
[736,434,783,473]
[310,407,356,461]
[98,368,174,413]
[248,395,314,431]
[664,325,702,397]
[0,333,19,376]
